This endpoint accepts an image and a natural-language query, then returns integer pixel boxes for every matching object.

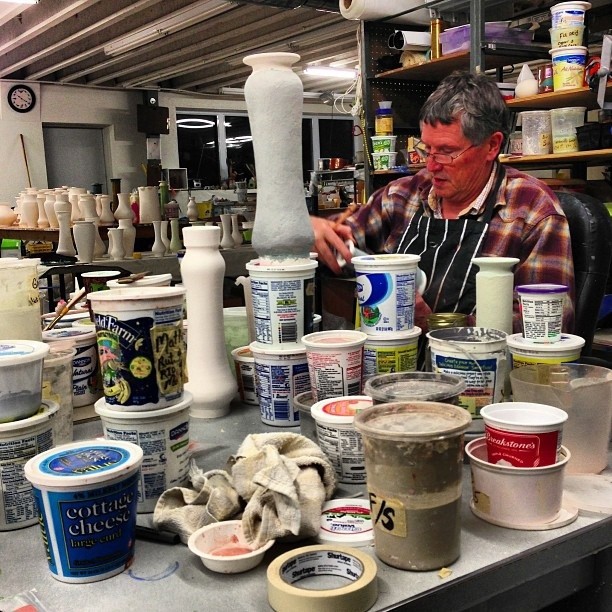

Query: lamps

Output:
[320,90,335,107]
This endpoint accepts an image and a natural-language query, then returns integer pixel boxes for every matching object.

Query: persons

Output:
[304,69,576,333]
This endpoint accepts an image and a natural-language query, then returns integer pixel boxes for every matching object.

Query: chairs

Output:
[38,266,133,312]
[556,192,612,360]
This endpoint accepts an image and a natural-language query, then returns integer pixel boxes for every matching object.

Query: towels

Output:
[153,432,335,549]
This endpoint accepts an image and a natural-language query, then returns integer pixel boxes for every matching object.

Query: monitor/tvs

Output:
[135,102,171,135]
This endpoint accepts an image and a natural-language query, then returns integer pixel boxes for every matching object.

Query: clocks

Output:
[8,84,36,112]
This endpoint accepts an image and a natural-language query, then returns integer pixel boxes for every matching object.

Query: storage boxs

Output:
[439,27,533,56]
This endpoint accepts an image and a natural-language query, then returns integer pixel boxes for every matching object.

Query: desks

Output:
[0,403,612,612]
[47,244,258,281]
[2,225,154,242]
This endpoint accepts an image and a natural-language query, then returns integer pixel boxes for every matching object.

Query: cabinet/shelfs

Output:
[476,0,612,164]
[163,168,189,190]
[360,0,476,172]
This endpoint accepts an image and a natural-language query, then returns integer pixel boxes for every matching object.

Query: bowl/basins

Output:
[465,439,570,521]
[188,520,274,574]
[510,362,611,476]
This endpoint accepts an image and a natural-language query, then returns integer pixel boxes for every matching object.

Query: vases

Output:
[153,221,165,257]
[188,197,198,220]
[181,226,237,417]
[243,53,313,258]
[118,219,136,256]
[189,221,206,226]
[73,221,95,263]
[231,215,243,245]
[16,186,56,228]
[108,229,113,260]
[219,215,235,250]
[170,218,182,253]
[85,217,105,257]
[206,222,213,226]
[162,221,170,255]
[56,211,76,256]
[111,228,126,261]
[56,186,115,222]
[472,257,521,334]
[216,222,223,241]
[114,192,135,219]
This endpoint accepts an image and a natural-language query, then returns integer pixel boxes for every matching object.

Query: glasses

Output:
[413,140,474,165]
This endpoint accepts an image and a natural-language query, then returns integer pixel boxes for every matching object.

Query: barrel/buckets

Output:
[246,258,319,350]
[426,326,508,432]
[519,111,552,155]
[40,346,77,445]
[249,341,312,427]
[506,332,585,418]
[362,369,467,407]
[547,26,586,48]
[548,107,587,154]
[0,257,41,342]
[105,273,174,288]
[231,346,259,405]
[42,328,97,408]
[309,396,372,485]
[0,399,60,532]
[87,287,187,412]
[547,46,588,93]
[548,0,592,28]
[25,438,144,585]
[516,283,570,344]
[353,400,473,571]
[301,329,367,402]
[351,254,421,334]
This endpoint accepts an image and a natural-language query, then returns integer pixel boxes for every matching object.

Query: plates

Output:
[468,486,579,529]
